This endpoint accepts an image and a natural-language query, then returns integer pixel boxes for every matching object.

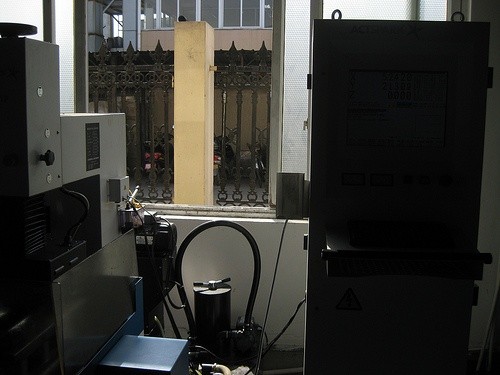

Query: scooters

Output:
[144,124,174,181]
[211,135,234,185]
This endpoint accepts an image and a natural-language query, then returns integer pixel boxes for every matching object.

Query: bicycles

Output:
[248,142,266,188]
